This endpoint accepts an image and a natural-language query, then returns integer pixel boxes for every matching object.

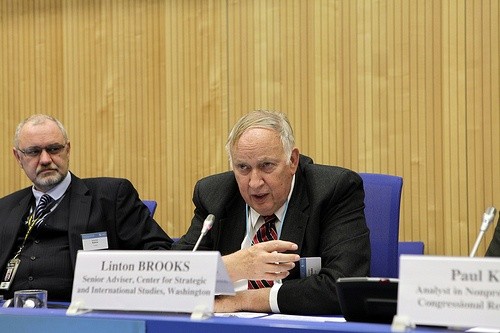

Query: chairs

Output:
[358,172,427,277]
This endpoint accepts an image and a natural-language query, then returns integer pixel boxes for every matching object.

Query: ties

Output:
[248,215,278,290]
[27,193,52,239]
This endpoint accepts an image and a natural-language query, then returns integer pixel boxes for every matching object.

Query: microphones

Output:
[193,213,216,253]
[470,207,497,258]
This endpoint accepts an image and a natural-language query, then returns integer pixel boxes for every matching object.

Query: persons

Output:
[172,110,370,315]
[0,114,173,302]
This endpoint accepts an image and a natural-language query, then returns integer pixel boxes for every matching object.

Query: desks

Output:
[0,302,442,333]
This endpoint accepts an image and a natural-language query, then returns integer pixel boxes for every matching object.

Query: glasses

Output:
[17,141,67,158]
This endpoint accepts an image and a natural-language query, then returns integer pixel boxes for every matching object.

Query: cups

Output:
[14,290,47,309]
